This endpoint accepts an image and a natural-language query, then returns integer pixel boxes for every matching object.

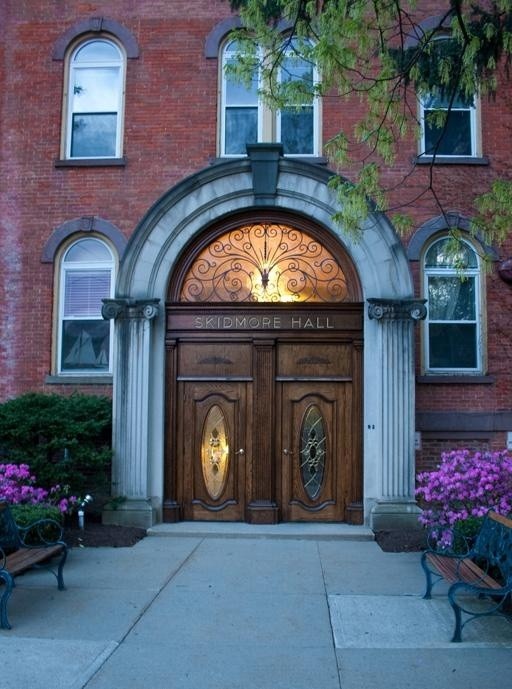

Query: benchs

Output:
[420,507,512,643]
[0,498,68,630]
